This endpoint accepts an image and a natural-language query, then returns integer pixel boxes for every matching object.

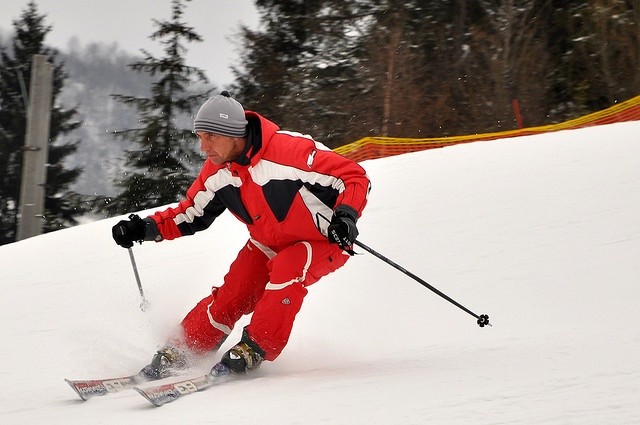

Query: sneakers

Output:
[152,347,187,377]
[221,342,264,373]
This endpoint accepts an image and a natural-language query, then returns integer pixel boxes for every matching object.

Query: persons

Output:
[112,97,370,375]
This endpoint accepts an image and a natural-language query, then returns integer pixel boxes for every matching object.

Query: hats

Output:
[194,91,249,138]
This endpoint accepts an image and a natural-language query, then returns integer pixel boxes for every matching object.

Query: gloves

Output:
[112,214,163,249]
[328,204,359,256]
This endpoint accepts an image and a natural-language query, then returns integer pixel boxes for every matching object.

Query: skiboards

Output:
[64,374,239,406]
